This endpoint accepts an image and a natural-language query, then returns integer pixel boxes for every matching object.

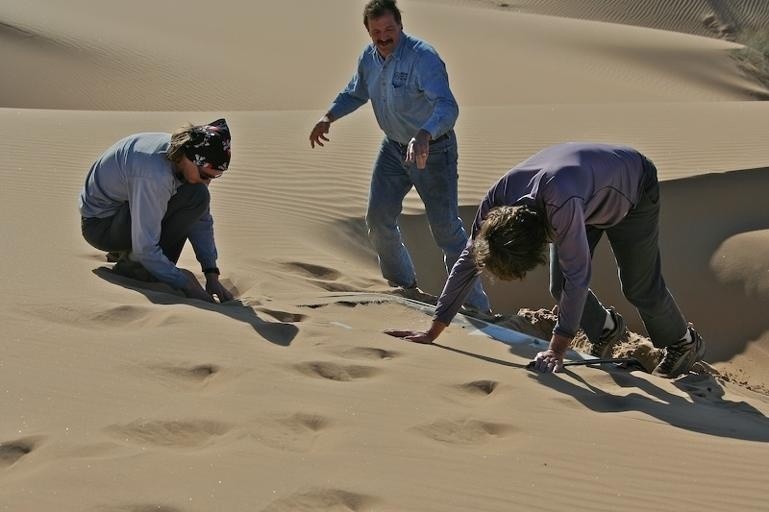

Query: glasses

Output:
[198,165,216,180]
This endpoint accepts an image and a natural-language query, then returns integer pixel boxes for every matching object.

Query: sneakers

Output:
[652,322,707,380]
[107,250,163,284]
[590,305,625,360]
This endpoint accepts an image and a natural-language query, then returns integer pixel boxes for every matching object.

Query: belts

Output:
[430,131,453,145]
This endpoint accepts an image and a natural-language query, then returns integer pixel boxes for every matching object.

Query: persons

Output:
[77,114,236,304]
[380,139,708,380]
[305,1,495,318]
